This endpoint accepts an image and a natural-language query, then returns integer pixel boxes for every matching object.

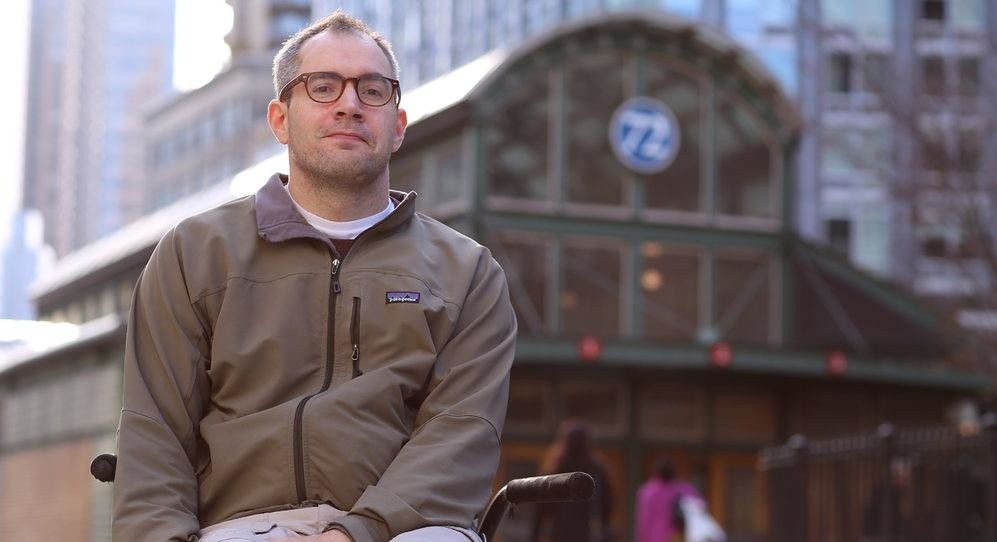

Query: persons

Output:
[635,461,705,542]
[111,11,519,542]
[527,418,615,542]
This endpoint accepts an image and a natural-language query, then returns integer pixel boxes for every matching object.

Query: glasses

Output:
[275,71,402,108]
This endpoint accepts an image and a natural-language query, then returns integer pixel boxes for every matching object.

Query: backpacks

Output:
[674,491,727,542]
